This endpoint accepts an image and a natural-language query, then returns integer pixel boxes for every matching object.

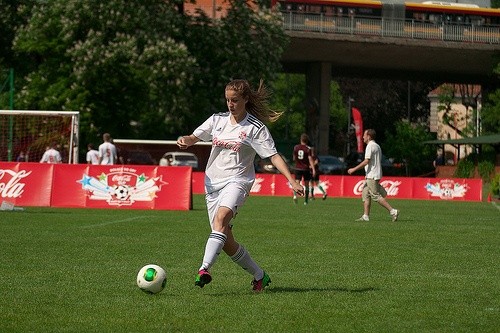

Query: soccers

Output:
[137,264,167,294]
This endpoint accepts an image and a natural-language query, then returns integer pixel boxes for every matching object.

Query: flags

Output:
[352,106,364,153]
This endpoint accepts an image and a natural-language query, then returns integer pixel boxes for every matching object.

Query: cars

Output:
[317,154,347,174]
[158,152,198,170]
[254,153,292,173]
[347,152,395,176]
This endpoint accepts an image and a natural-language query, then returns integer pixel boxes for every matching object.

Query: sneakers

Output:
[252,271,272,291]
[390,209,400,222]
[195,269,212,288]
[355,217,367,221]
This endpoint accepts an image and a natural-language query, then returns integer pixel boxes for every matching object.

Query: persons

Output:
[344,143,360,176]
[293,133,327,205]
[98,133,118,165]
[16,151,26,162]
[39,142,63,164]
[176,79,305,292]
[86,144,99,165]
[347,129,400,222]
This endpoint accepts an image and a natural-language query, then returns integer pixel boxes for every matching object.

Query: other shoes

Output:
[304,203,307,205]
[310,198,315,201]
[323,193,327,199]
[293,196,297,204]
